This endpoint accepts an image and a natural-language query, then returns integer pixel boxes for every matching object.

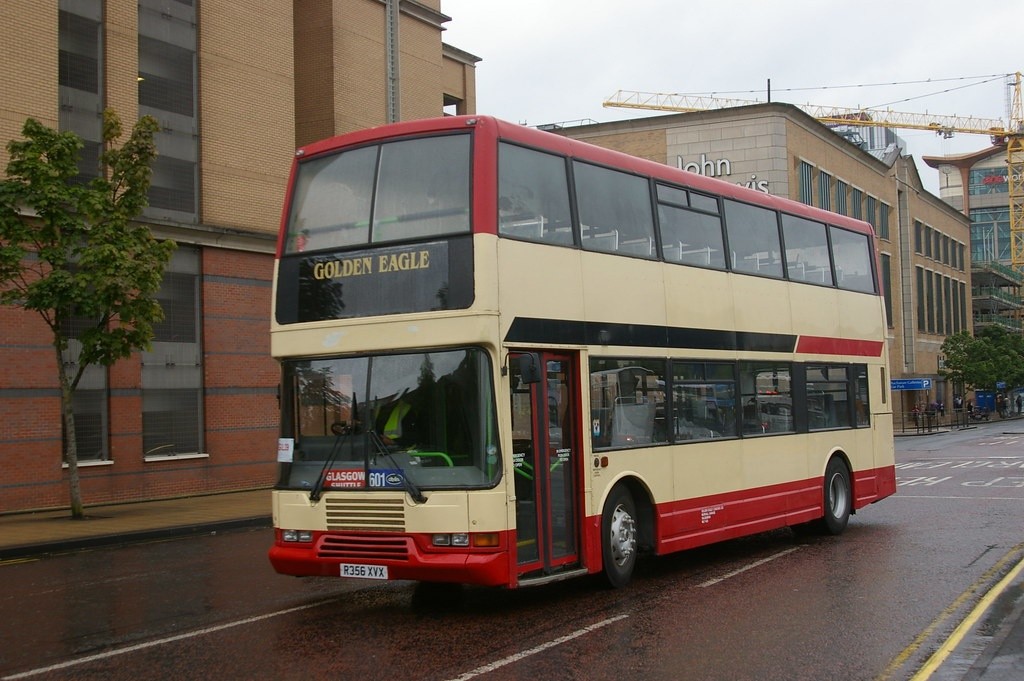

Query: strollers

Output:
[673,382,744,427]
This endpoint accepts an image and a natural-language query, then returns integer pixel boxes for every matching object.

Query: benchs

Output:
[804,266,824,283]
[759,257,782,276]
[734,252,759,272]
[824,265,844,286]
[710,250,736,269]
[787,261,806,280]
[543,222,583,245]
[617,234,651,256]
[651,240,683,261]
[501,215,543,238]
[682,245,711,265]
[583,228,618,251]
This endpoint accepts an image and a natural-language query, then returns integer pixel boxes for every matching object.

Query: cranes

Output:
[602,71,1024,327]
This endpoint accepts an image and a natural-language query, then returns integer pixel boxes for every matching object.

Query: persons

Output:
[953,396,973,422]
[912,400,944,426]
[984,395,1023,421]
[369,371,423,453]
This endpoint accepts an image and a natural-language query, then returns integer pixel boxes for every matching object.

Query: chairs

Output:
[706,400,724,427]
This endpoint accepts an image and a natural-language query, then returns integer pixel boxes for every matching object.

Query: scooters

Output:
[975,404,990,421]
[967,410,974,420]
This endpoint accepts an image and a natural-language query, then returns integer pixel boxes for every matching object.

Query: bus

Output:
[269,115,899,594]
